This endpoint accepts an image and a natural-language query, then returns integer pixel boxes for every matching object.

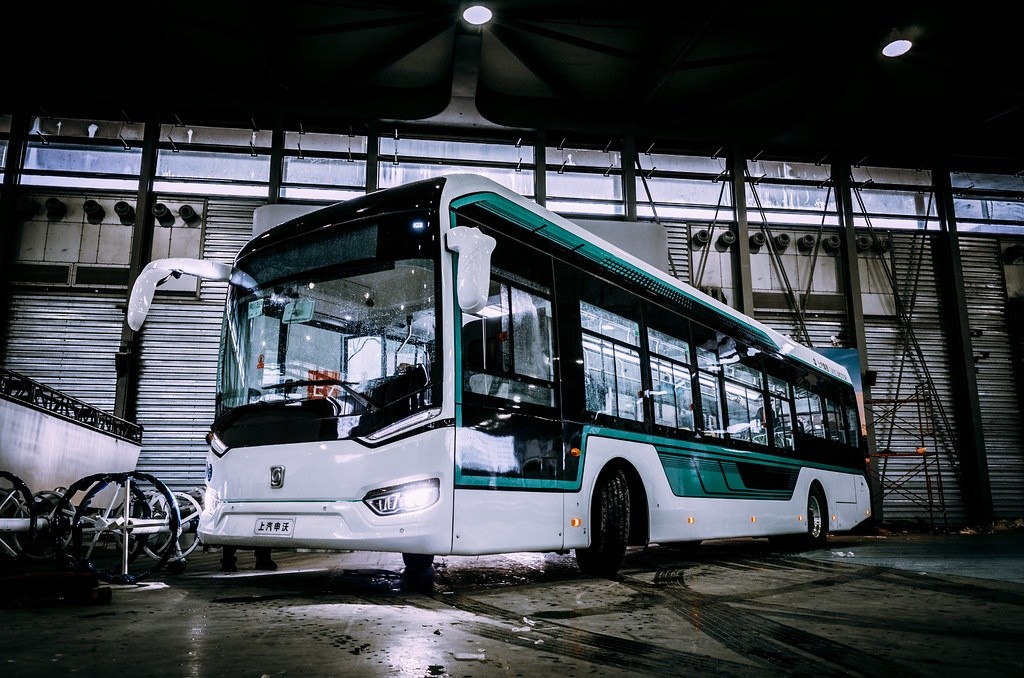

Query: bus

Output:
[127,173,874,571]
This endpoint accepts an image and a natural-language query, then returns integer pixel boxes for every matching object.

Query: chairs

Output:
[602,391,845,450]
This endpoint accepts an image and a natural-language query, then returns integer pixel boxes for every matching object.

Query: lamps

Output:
[881,27,913,58]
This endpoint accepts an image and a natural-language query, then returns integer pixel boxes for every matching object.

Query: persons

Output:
[219,544,279,572]
[734,388,782,432]
